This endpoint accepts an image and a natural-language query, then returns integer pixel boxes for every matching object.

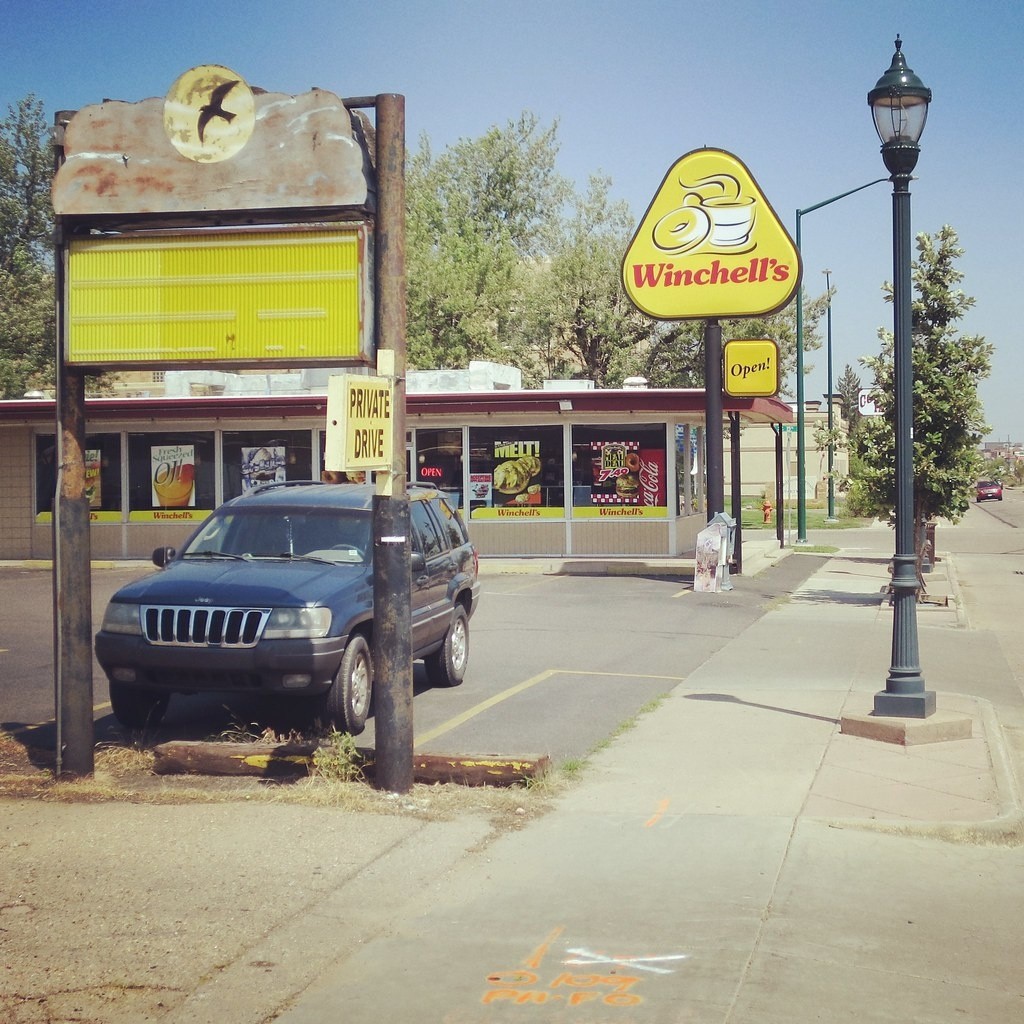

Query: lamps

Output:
[288,433,296,463]
[557,400,574,411]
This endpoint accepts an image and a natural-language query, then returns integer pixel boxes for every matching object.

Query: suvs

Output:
[93,480,480,736]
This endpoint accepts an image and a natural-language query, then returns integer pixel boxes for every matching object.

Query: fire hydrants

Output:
[762,499,773,525]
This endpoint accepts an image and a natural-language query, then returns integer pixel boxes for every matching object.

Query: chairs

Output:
[243,518,290,557]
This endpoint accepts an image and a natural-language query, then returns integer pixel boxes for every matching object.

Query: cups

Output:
[154,470,193,506]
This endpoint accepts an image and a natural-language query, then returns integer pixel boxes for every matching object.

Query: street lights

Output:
[795,174,922,544]
[864,29,940,722]
[821,269,835,519]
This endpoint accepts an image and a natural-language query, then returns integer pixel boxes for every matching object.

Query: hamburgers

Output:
[84,475,96,503]
[616,473,639,498]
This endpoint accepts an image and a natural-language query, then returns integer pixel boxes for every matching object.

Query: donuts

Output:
[625,453,640,472]
[321,470,341,484]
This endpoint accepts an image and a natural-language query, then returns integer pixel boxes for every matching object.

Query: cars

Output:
[997,480,1003,489]
[975,479,1002,503]
